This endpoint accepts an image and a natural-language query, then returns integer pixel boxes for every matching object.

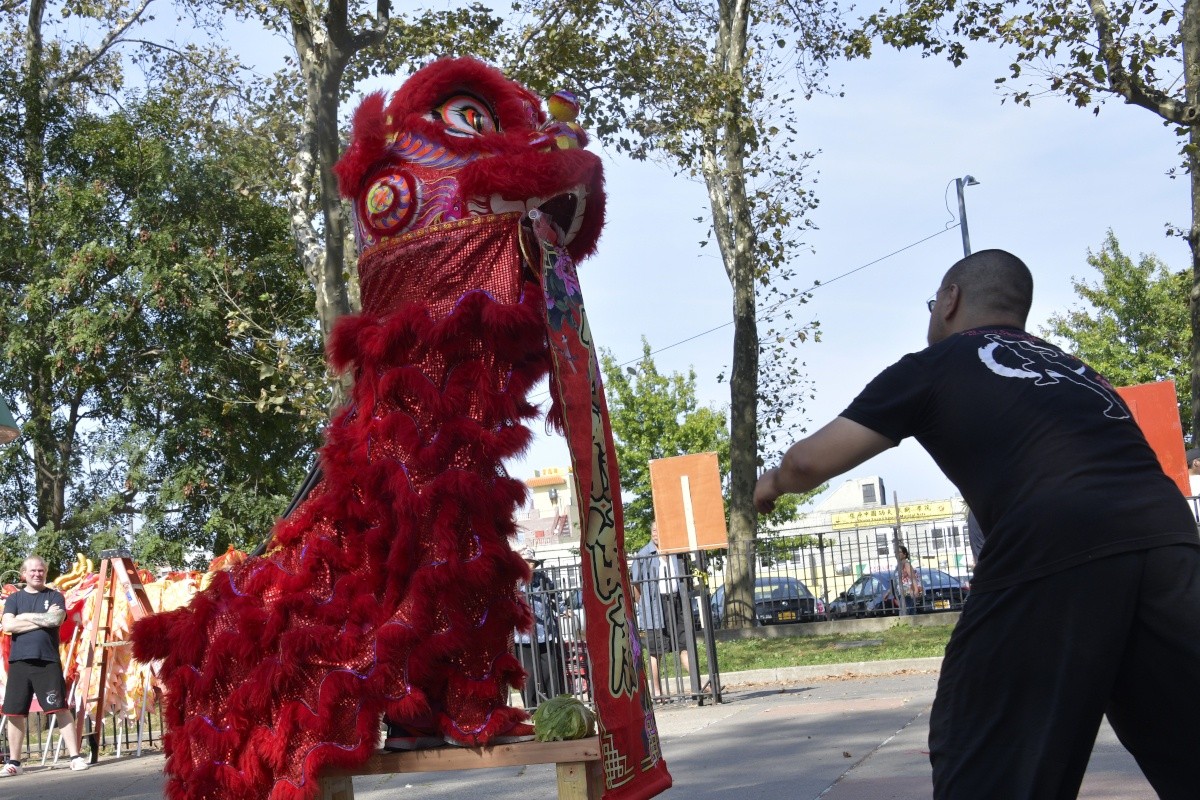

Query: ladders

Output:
[76,547,166,763]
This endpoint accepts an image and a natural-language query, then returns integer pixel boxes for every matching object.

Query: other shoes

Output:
[650,697,674,703]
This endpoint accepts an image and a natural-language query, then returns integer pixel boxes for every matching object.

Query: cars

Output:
[562,589,587,643]
[829,568,971,620]
[710,577,829,625]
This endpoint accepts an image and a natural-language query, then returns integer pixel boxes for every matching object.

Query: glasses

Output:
[925,285,950,313]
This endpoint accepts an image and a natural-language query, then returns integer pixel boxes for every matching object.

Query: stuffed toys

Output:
[128,57,607,800]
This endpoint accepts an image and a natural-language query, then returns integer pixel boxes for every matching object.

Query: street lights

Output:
[951,174,980,261]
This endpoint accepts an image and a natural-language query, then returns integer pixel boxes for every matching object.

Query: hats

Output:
[516,546,540,569]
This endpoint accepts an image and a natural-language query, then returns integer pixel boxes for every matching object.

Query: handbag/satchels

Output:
[896,570,925,597]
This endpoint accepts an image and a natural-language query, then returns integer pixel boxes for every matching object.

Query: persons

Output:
[517,548,574,716]
[886,545,920,614]
[753,249,1200,800]
[0,554,88,779]
[632,520,711,703]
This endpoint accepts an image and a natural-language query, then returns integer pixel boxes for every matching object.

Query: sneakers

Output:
[70,757,89,770]
[0,762,23,777]
